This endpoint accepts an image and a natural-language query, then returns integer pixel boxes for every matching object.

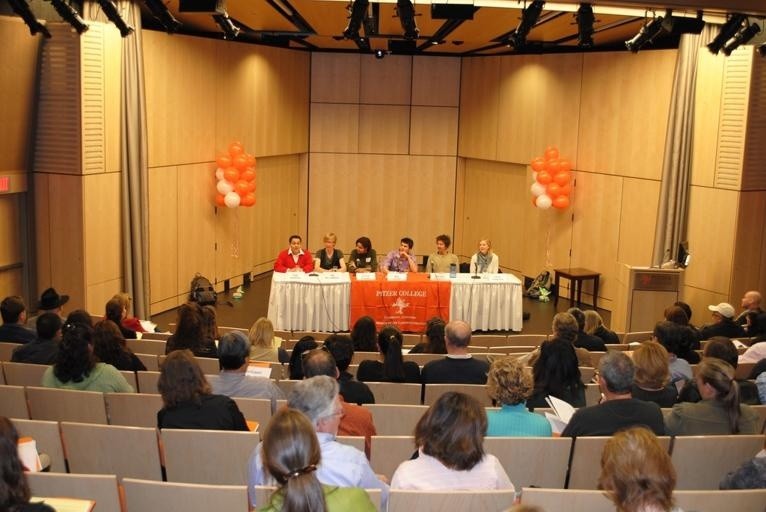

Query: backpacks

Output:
[190,272,218,307]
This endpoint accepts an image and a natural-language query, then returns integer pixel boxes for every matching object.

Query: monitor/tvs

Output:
[677,241,691,268]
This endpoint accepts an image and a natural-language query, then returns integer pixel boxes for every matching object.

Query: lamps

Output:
[10,0,761,58]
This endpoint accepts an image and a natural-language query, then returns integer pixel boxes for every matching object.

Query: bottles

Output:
[297,262,457,280]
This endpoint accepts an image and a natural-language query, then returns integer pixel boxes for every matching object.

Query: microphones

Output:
[659,249,670,268]
[471,262,480,278]
[428,260,434,276]
[310,261,319,276]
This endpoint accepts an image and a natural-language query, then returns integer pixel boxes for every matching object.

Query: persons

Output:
[389,391,517,502]
[347,236,378,273]
[381,238,419,273]
[483,290,766,436]
[469,239,499,274]
[248,374,390,512]
[0,415,56,512]
[273,235,315,273]
[0,416,30,471]
[425,235,460,274]
[1,286,284,437]
[312,232,347,273]
[598,425,683,512]
[718,439,766,490]
[254,406,378,512]
[293,314,489,434]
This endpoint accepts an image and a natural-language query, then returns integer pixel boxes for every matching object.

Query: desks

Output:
[267,270,523,333]
[554,267,600,310]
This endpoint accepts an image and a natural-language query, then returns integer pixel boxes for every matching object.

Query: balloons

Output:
[530,144,574,210]
[213,138,258,209]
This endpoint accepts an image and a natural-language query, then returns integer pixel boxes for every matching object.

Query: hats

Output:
[708,302,736,318]
[34,287,69,310]
